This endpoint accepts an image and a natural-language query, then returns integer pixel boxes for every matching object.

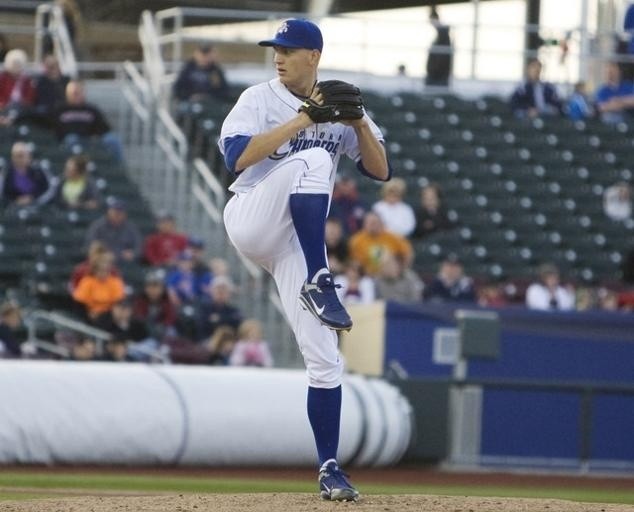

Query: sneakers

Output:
[318,461,358,502]
[296,272,356,331]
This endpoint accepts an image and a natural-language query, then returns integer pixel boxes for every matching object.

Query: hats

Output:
[107,196,234,297]
[259,17,325,53]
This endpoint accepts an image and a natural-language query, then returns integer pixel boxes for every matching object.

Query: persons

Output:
[217,19,394,501]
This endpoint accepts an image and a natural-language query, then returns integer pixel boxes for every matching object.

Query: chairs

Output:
[0,115,160,345]
[355,88,633,303]
[185,83,241,195]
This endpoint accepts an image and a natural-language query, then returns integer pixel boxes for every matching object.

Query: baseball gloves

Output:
[298,81,365,124]
